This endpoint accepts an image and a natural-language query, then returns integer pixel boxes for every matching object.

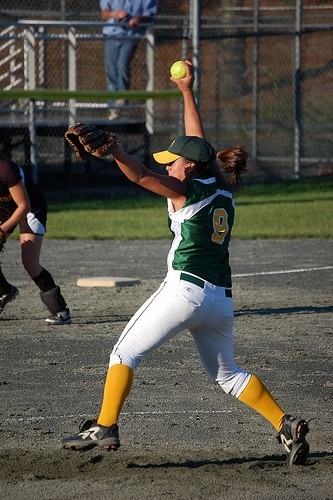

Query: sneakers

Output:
[45,307,72,325]
[61,420,121,451]
[278,413,311,466]
[0,285,19,314]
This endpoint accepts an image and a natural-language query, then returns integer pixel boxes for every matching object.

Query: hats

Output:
[153,135,215,165]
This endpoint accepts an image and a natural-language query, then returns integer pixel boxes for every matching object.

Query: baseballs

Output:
[169,60,186,79]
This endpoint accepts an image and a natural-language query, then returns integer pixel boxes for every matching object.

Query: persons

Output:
[0,153,71,325]
[60,62,309,467]
[98,0,160,120]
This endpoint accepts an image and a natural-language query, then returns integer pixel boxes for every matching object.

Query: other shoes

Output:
[116,99,127,108]
[109,109,120,120]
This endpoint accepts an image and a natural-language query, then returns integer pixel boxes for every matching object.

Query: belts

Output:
[181,272,232,298]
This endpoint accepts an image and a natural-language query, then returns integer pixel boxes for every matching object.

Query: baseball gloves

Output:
[64,122,120,163]
[0,226,7,253]
